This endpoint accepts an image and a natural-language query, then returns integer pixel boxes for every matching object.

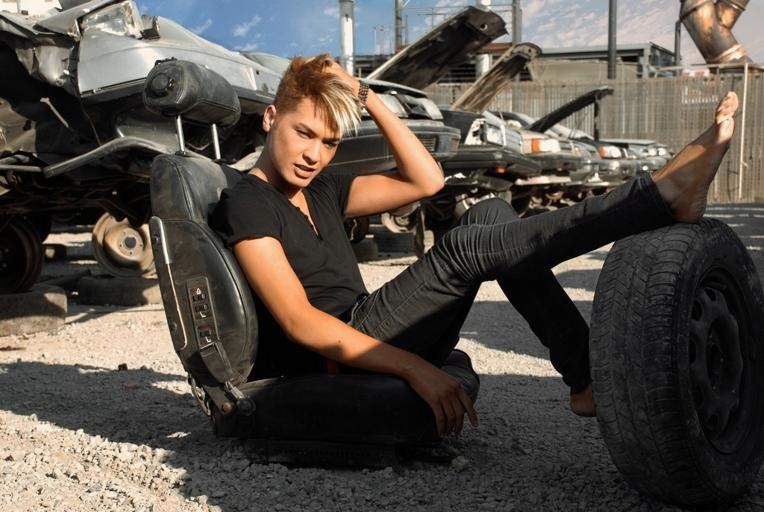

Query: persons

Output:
[209,53,739,440]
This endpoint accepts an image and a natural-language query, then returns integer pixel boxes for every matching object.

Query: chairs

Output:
[140,59,481,470]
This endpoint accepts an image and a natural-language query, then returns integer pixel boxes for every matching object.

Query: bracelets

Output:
[357,81,370,110]
